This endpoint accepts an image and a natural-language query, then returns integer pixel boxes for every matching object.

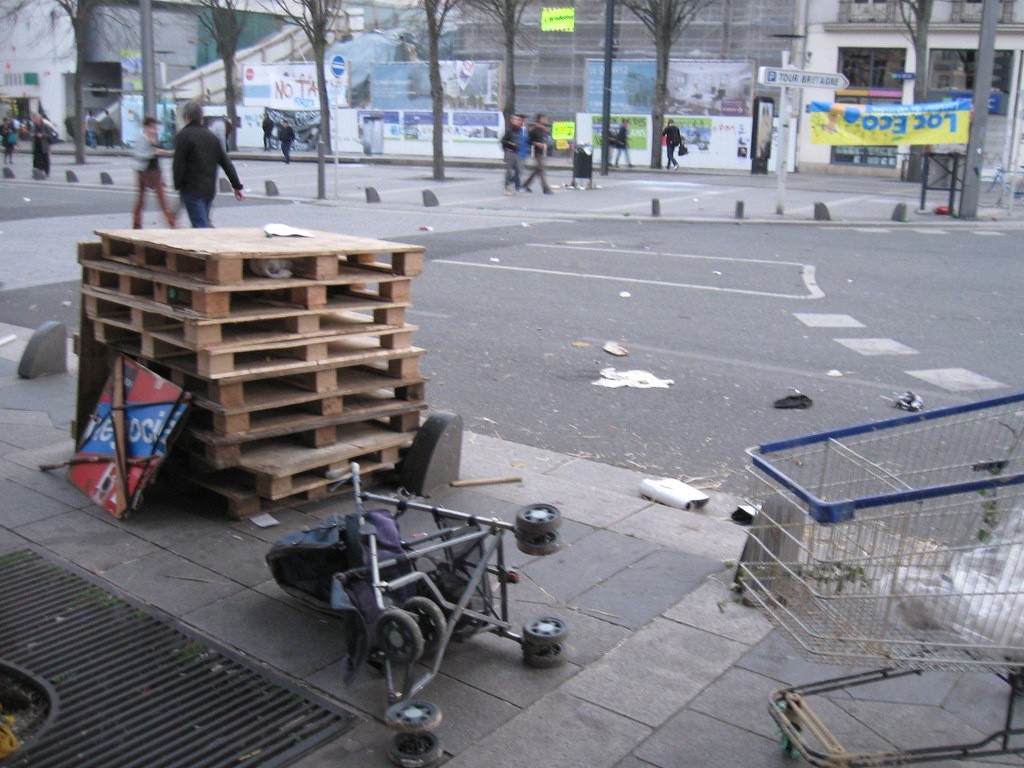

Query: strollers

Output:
[266,463,568,768]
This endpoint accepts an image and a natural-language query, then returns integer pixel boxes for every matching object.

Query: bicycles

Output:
[976,166,1024,208]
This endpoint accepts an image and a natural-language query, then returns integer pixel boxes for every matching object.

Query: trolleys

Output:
[738,393,1024,768]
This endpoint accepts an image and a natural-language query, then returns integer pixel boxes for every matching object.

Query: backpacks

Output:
[667,127,680,146]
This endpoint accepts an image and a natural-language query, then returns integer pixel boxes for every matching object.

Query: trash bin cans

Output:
[572,143,596,186]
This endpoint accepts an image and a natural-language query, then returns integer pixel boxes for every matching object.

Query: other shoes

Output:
[615,164,620,168]
[543,186,553,194]
[523,183,531,192]
[626,164,632,168]
[672,164,679,171]
[664,165,669,171]
[503,188,512,196]
[513,189,522,195]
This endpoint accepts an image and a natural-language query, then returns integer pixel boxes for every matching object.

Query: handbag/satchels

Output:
[7,131,17,145]
[678,145,688,156]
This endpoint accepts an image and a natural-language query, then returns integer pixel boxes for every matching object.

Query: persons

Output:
[608,118,634,168]
[279,120,293,164]
[0,115,27,165]
[132,115,178,228]
[29,114,58,177]
[662,119,683,171]
[173,102,245,228]
[86,110,97,147]
[262,113,275,150]
[501,112,556,196]
[101,110,115,148]
